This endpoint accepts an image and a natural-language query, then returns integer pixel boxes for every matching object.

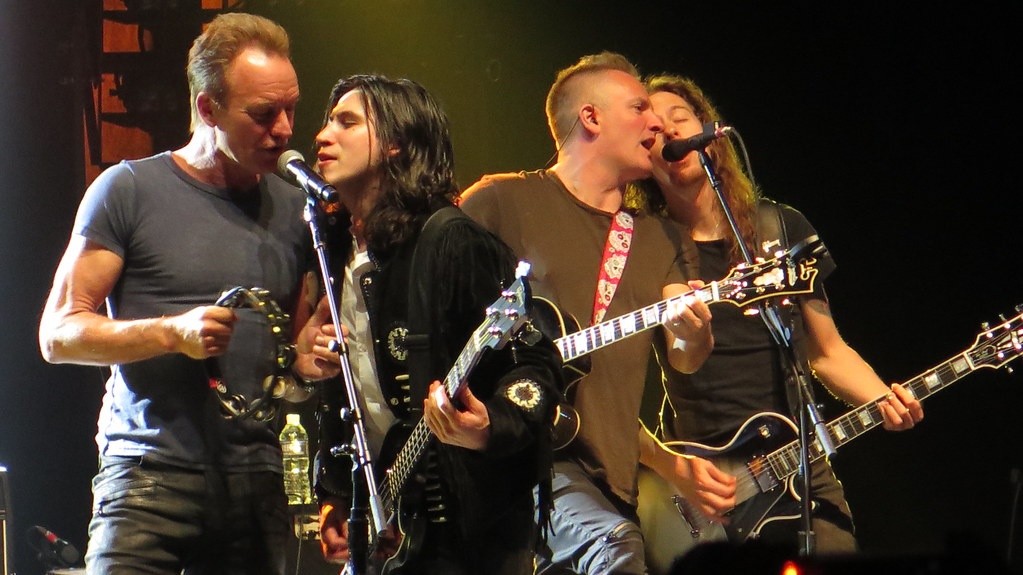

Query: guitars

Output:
[526,240,821,460]
[630,301,1023,575]
[340,272,538,575]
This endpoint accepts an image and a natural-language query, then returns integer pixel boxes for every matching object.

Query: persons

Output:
[37,14,349,575]
[458,51,924,575]
[319,75,564,575]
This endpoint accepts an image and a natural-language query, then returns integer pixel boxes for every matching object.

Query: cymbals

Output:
[193,282,300,428]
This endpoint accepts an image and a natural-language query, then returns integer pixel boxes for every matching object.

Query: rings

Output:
[670,323,681,329]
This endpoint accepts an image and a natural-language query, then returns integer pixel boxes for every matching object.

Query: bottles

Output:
[278,413,312,505]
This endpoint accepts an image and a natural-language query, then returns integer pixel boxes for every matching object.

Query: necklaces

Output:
[709,217,725,240]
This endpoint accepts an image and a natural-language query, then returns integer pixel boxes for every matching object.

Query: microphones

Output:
[36,525,79,563]
[277,149,339,203]
[662,121,730,162]
